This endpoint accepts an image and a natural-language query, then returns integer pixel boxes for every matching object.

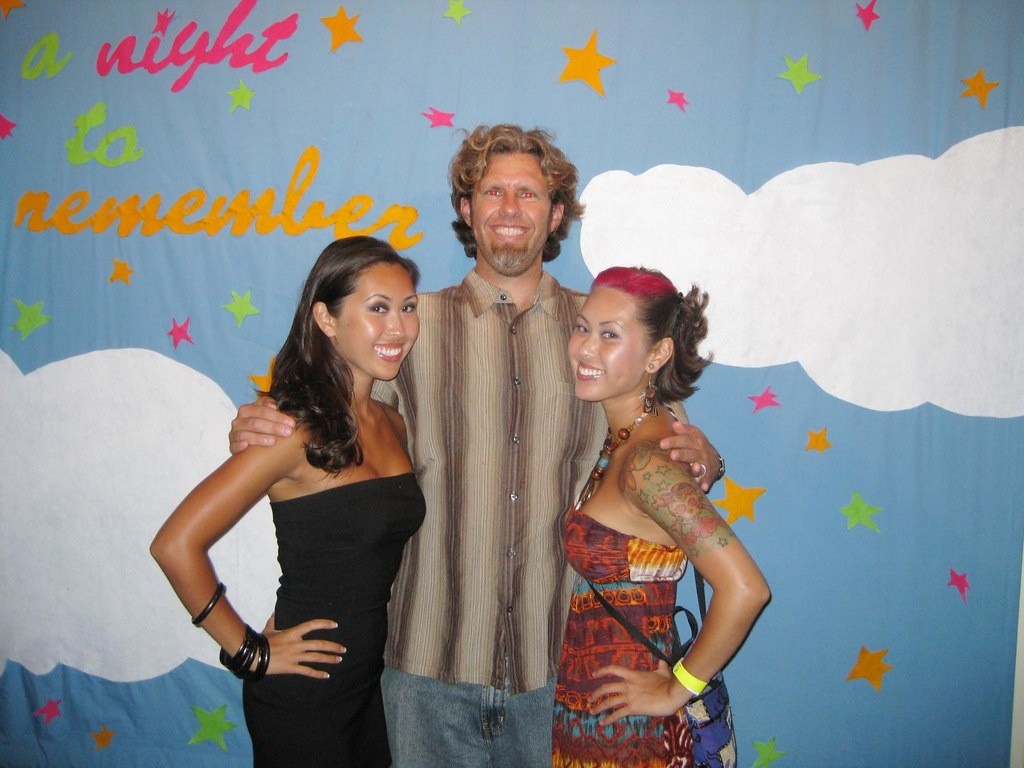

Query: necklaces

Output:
[578,412,648,503]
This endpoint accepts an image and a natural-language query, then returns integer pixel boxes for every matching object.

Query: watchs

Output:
[718,457,725,477]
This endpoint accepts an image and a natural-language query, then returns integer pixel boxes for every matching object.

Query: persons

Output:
[149,123,772,768]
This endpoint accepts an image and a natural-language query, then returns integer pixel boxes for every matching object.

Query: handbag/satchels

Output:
[663,607,737,768]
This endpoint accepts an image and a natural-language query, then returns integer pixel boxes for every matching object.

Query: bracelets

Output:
[220,624,270,682]
[192,582,223,624]
[673,657,710,696]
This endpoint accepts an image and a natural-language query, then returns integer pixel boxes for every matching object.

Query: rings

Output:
[698,464,706,479]
